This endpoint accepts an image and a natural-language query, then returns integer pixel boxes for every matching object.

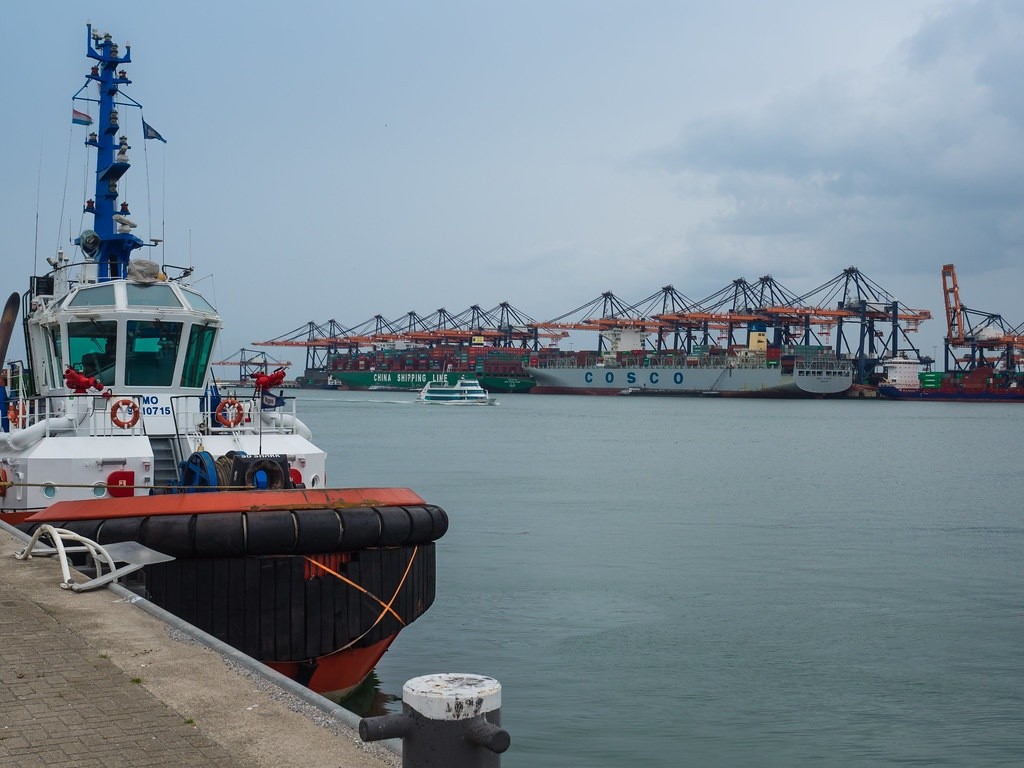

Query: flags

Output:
[142,120,167,144]
[72,109,93,126]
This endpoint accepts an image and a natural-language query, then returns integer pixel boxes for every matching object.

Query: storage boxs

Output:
[307,378,314,384]
[918,371,1024,388]
[326,345,846,373]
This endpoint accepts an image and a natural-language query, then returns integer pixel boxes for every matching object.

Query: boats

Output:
[416,378,496,407]
[516,268,932,398]
[619,387,640,396]
[0,21,451,701]
[295,374,343,391]
[697,390,720,398]
[250,263,538,392]
[879,264,1024,404]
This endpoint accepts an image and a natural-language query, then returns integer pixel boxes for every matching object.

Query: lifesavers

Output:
[216,400,244,427]
[0,468,7,498]
[110,399,140,428]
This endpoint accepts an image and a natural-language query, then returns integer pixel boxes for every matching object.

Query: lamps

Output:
[81,231,100,261]
